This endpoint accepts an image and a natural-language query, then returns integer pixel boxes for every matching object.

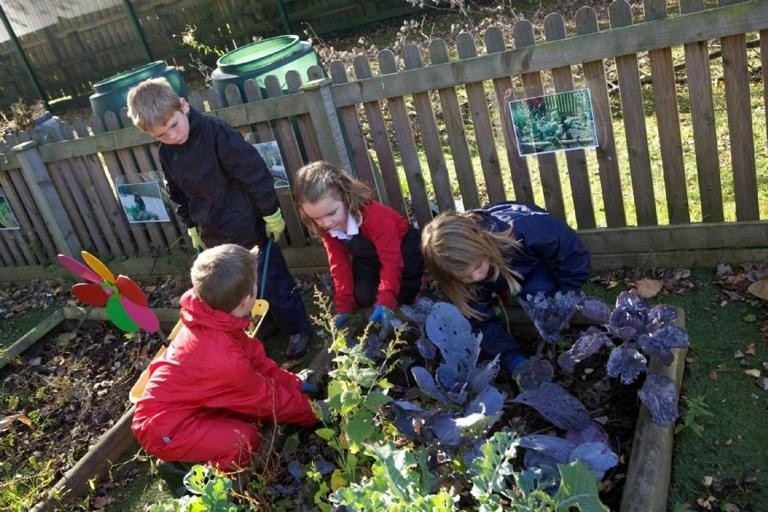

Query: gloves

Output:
[298,368,318,392]
[262,206,286,243]
[367,304,398,328]
[187,226,208,250]
[333,311,353,326]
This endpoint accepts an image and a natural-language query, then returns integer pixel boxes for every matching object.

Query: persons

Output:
[295,160,425,339]
[418,202,590,367]
[127,79,312,357]
[132,244,318,494]
